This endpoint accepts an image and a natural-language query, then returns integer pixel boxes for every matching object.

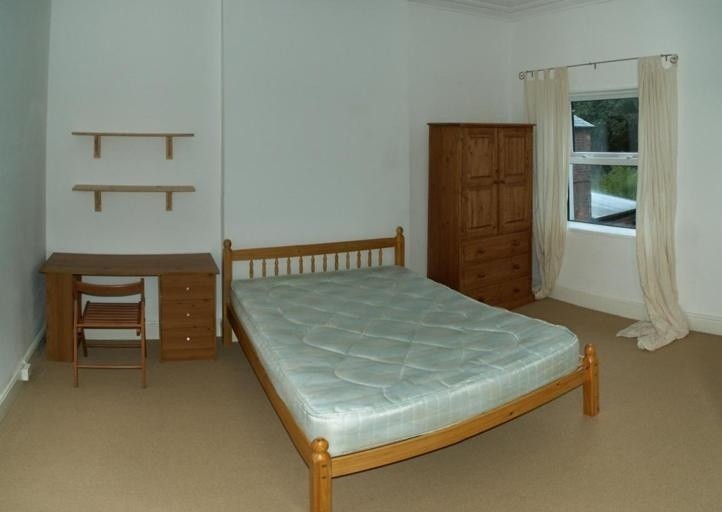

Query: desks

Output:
[42,250,218,364]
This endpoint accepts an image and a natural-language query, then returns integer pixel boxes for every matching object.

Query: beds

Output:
[220,228,601,512]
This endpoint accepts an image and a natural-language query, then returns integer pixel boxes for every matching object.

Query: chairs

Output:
[61,275,150,389]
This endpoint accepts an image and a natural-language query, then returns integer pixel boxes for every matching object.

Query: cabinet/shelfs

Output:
[426,120,540,308]
[64,123,196,211]
